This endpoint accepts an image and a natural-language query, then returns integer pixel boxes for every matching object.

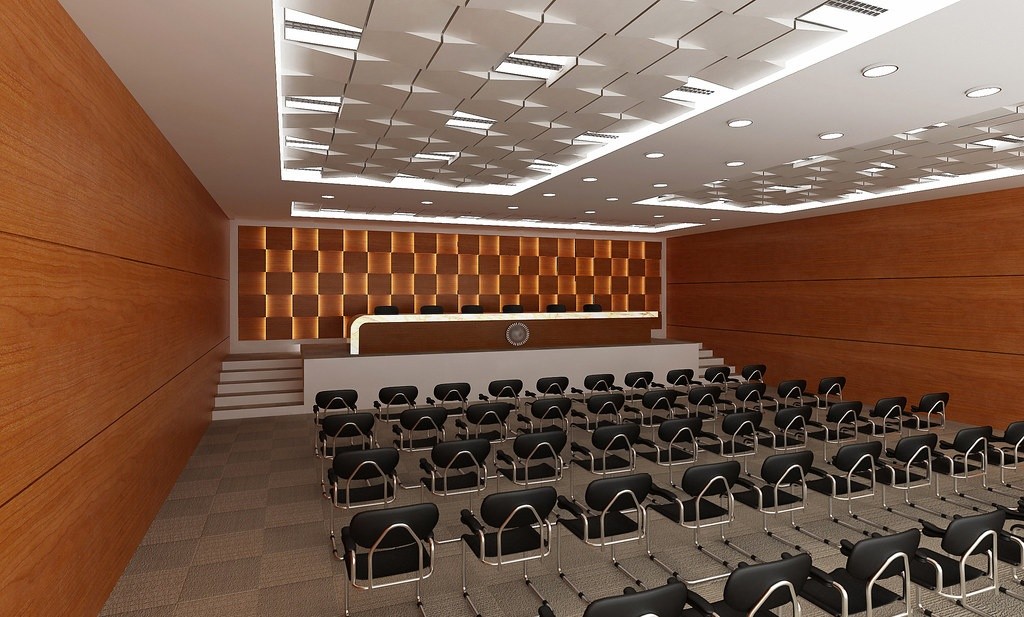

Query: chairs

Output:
[312,364,1024,617]
[374,304,601,314]
